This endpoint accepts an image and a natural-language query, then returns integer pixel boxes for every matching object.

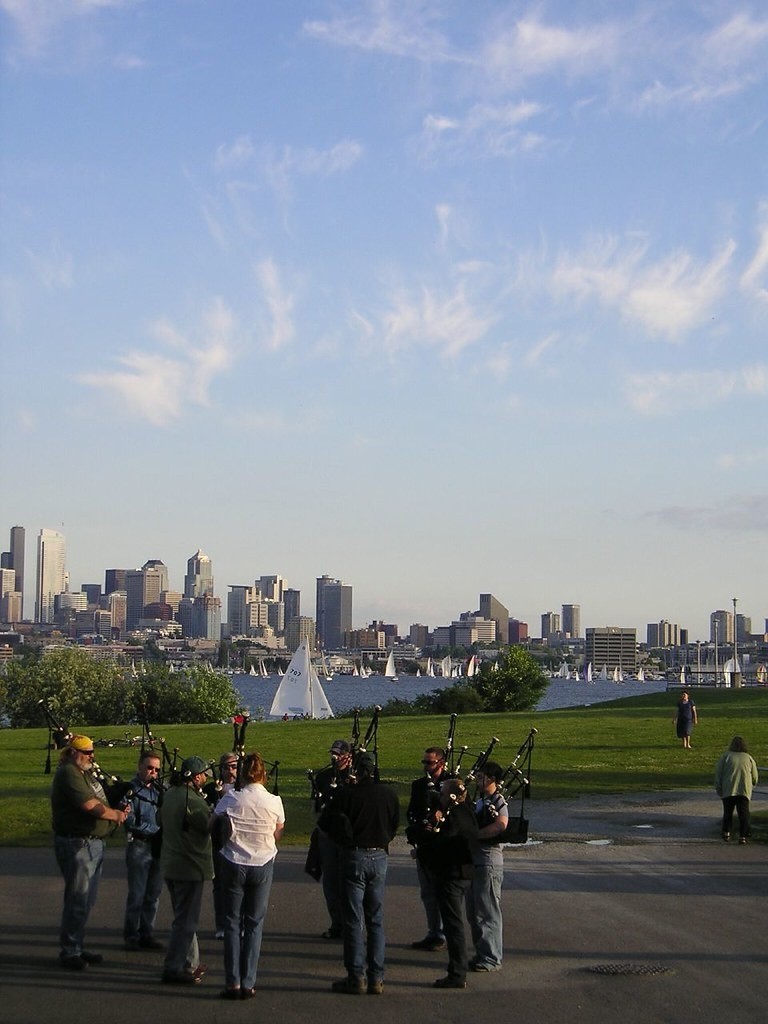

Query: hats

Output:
[471,762,503,785]
[327,740,350,755]
[181,756,207,773]
[66,734,93,750]
[351,752,374,767]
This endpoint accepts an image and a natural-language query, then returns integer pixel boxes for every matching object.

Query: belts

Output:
[337,848,385,852]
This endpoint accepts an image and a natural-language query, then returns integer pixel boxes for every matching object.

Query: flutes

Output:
[231,710,280,796]
[418,736,499,838]
[471,727,538,842]
[141,702,182,795]
[421,712,468,822]
[184,753,244,808]
[349,705,382,785]
[44,703,135,835]
[305,755,357,809]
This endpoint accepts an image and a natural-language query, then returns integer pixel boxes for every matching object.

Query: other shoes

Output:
[331,972,367,995]
[424,938,448,951]
[721,833,731,842]
[468,956,488,972]
[738,839,750,845]
[411,936,435,949]
[58,925,257,1000]
[431,970,466,988]
[322,925,344,939]
[367,976,384,994]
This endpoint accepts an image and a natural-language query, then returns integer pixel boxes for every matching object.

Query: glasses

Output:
[420,760,441,766]
[74,749,95,755]
[142,763,161,772]
[219,765,238,770]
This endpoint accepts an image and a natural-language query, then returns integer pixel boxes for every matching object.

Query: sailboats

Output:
[113,648,665,685]
[269,635,335,720]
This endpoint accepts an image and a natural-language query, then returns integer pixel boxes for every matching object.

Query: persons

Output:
[305,740,400,994]
[405,747,509,988]
[282,712,310,721]
[50,734,131,971]
[671,690,698,748]
[714,737,758,845]
[119,752,285,999]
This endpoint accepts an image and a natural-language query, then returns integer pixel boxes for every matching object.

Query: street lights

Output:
[730,597,742,689]
[712,618,720,687]
[696,639,702,686]
